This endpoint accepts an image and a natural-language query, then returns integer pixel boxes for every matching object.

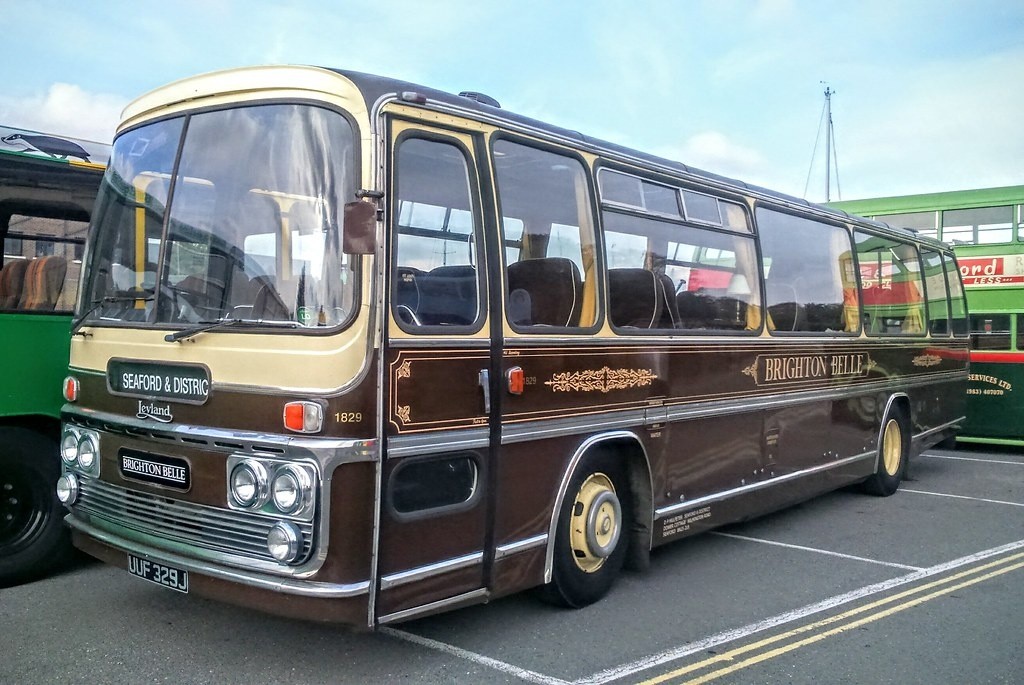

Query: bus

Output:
[54,64,969,628]
[793,185,1023,453]
[0,126,328,589]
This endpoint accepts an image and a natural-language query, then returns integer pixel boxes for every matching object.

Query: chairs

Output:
[7,240,945,340]
